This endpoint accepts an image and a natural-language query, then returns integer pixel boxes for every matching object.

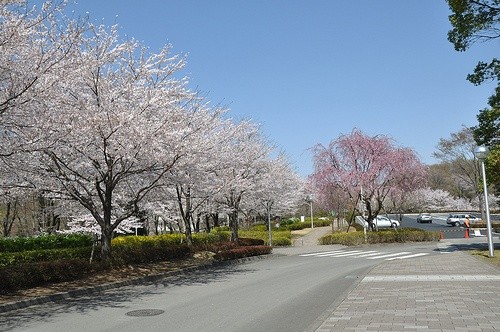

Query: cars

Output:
[474,213,482,222]
[365,215,400,230]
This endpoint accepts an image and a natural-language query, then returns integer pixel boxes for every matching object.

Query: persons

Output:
[464,214,471,237]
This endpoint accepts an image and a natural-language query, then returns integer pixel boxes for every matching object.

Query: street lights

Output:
[473,145,494,257]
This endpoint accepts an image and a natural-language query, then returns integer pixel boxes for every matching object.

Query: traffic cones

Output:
[440,229,444,239]
[464,228,469,238]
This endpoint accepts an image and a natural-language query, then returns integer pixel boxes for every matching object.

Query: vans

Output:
[447,214,477,227]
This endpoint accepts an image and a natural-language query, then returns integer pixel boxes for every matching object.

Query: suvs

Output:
[417,212,432,223]
